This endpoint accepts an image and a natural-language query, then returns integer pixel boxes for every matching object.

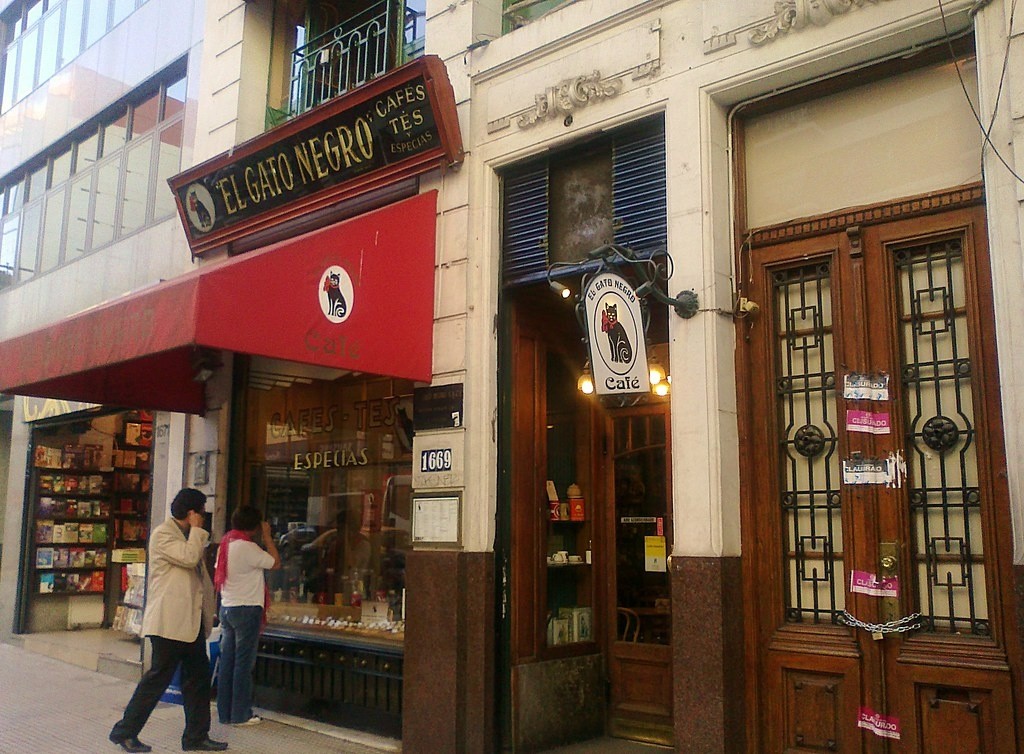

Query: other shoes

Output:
[232,715,264,728]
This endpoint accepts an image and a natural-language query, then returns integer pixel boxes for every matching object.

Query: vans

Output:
[380,475,413,558]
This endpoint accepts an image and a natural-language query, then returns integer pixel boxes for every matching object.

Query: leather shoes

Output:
[181,734,229,751]
[109,731,153,753]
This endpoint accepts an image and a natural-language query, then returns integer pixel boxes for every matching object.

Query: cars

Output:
[278,526,318,560]
[299,529,406,570]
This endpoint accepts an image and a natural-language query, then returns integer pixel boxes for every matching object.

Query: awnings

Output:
[0,188,438,413]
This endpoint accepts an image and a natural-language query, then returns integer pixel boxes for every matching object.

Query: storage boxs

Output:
[548,605,592,647]
[110,548,146,563]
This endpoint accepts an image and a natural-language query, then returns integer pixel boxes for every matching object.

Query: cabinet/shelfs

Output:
[547,518,594,659]
[112,417,153,549]
[18,466,116,635]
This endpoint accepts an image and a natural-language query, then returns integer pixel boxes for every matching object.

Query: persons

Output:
[216,506,280,726]
[380,536,406,622]
[302,510,370,623]
[109,488,228,753]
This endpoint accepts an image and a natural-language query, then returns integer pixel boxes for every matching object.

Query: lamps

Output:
[577,340,672,398]
[546,243,699,319]
